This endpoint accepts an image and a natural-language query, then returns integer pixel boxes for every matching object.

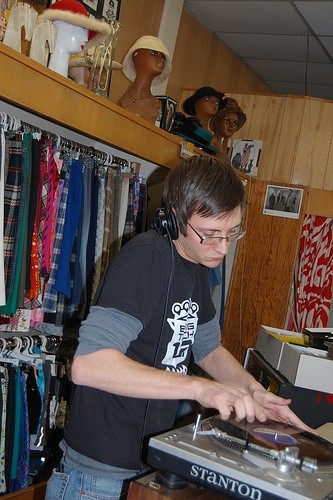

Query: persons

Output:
[117,35,164,127]
[41,0,91,79]
[209,97,247,155]
[239,143,250,172]
[184,87,227,145]
[44,155,320,500]
[269,190,297,212]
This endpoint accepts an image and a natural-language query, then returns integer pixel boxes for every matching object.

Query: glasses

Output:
[185,218,247,246]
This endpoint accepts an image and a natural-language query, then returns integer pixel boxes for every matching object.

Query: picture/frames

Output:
[231,137,263,177]
[263,185,303,219]
[154,95,168,129]
[164,97,178,130]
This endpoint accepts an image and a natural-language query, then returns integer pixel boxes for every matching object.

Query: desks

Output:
[124,471,237,500]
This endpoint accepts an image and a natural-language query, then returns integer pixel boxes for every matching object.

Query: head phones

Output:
[155,207,177,241]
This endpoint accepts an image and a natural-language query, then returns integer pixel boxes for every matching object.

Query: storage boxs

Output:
[256,324,308,369]
[281,342,333,395]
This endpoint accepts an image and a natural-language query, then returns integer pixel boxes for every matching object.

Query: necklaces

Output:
[128,90,152,104]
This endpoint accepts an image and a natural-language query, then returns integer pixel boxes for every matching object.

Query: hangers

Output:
[0,112,137,177]
[0,335,79,365]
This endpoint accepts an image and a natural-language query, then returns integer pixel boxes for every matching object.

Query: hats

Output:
[182,86,226,116]
[122,35,172,86]
[37,0,111,52]
[209,97,247,133]
[68,44,125,71]
[169,111,219,154]
